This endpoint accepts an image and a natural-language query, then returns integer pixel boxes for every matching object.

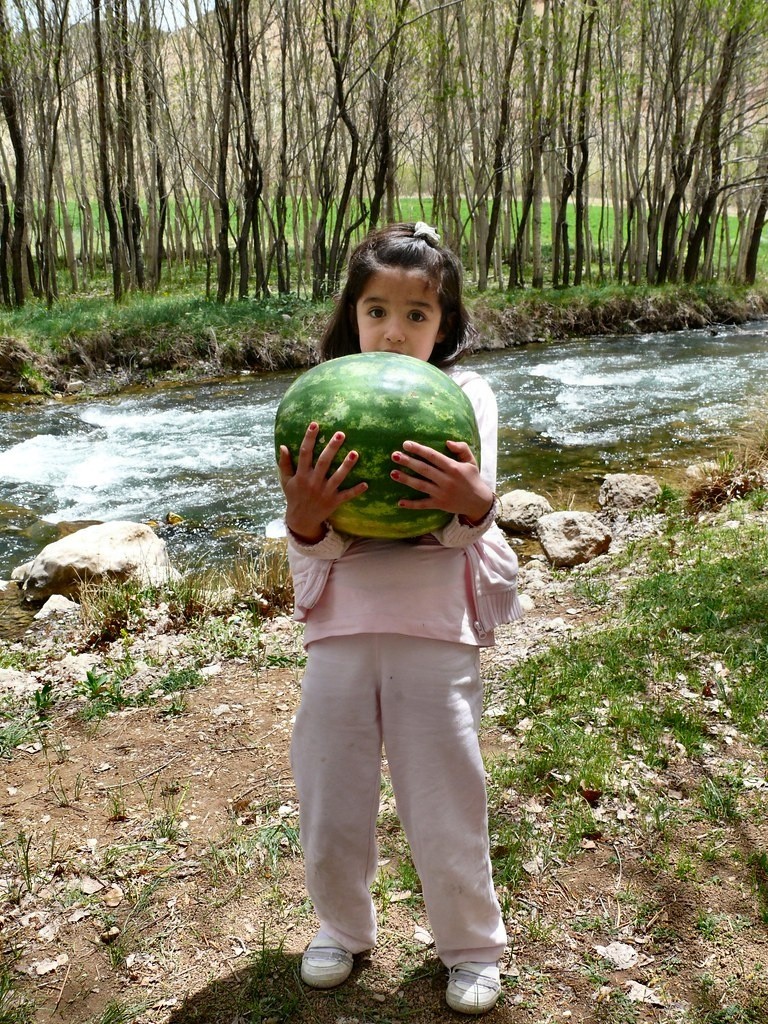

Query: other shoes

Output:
[300,929,354,988]
[445,962,502,1014]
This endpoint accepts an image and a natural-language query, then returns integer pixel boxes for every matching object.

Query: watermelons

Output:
[277,351,482,539]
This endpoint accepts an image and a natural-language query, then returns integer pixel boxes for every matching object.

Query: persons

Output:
[278,221,519,1015]
